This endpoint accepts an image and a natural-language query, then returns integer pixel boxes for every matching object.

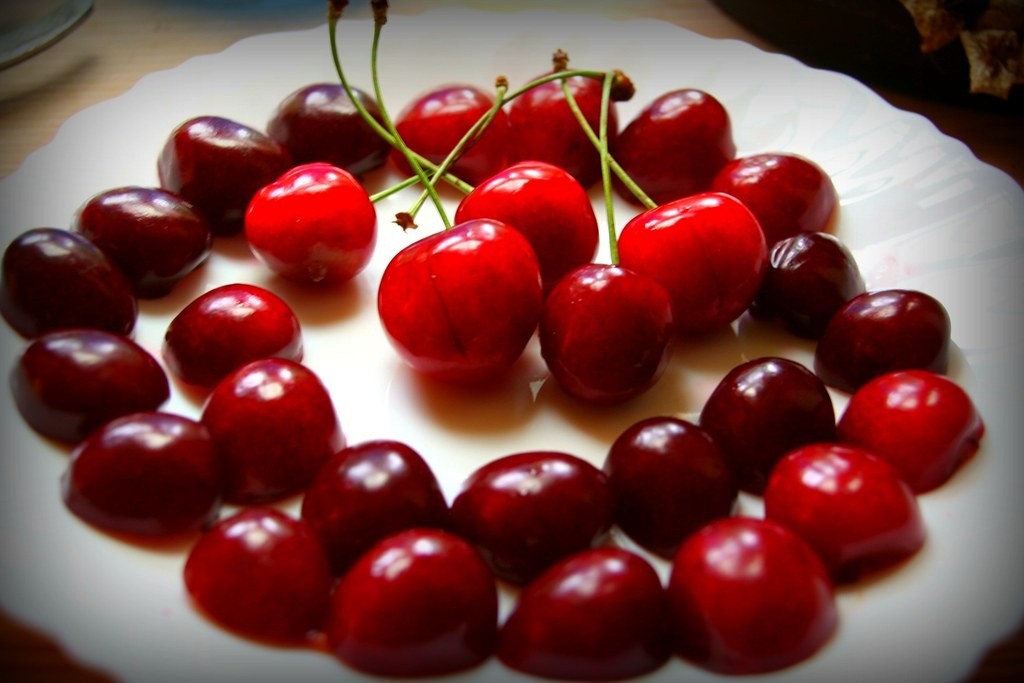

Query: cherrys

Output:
[0,1,984,682]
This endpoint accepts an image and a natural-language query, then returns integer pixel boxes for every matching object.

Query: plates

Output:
[0,7,1024,683]
